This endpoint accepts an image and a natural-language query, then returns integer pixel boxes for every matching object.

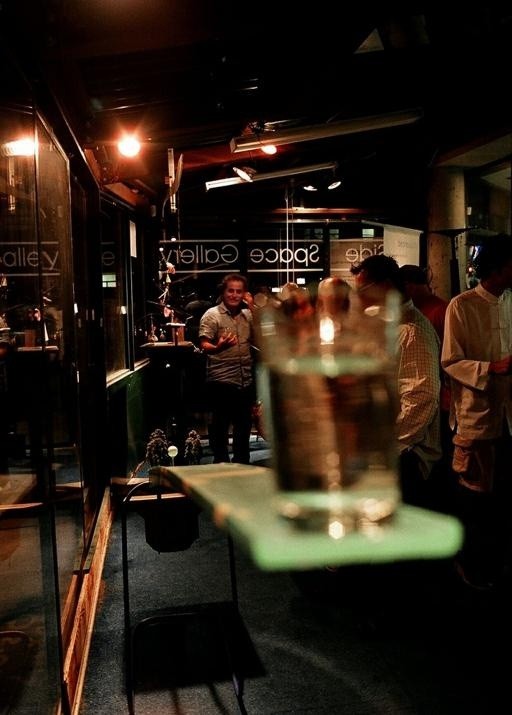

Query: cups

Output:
[250,287,405,542]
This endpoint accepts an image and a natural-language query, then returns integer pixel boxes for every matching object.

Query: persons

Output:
[183,246,511,504]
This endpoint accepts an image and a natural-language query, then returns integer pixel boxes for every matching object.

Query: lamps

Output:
[260,122,278,155]
[232,160,256,183]
[327,176,341,190]
